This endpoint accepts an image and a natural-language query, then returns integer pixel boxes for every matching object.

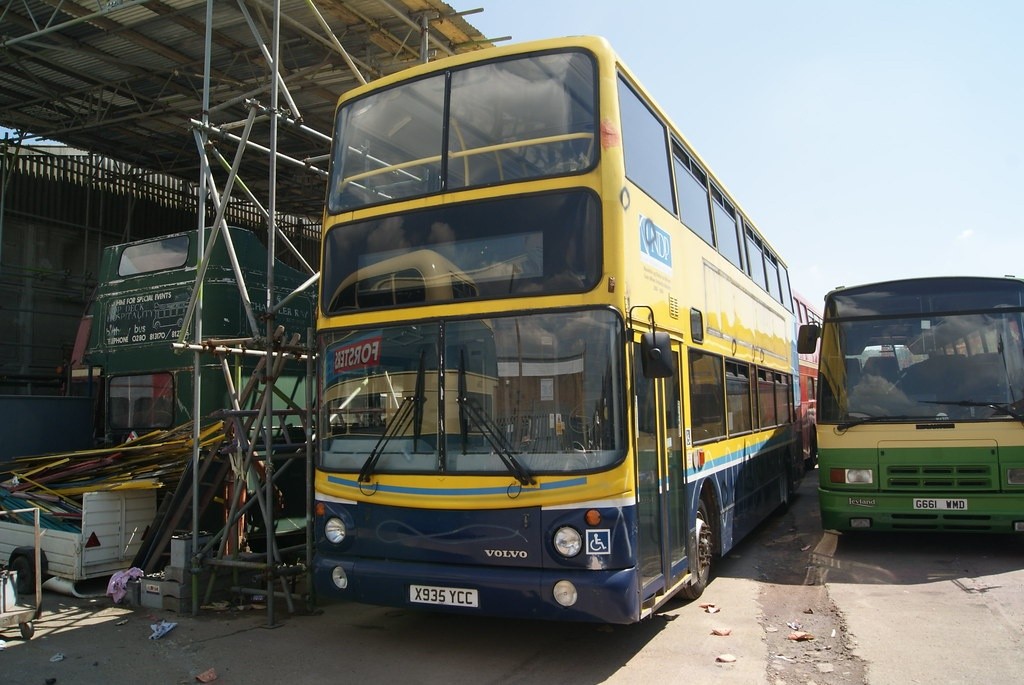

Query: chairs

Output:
[845,353,1010,411]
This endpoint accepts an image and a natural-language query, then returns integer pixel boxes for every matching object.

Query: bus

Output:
[797,273,1024,552]
[323,248,501,453]
[300,35,827,626]
[58,225,317,529]
